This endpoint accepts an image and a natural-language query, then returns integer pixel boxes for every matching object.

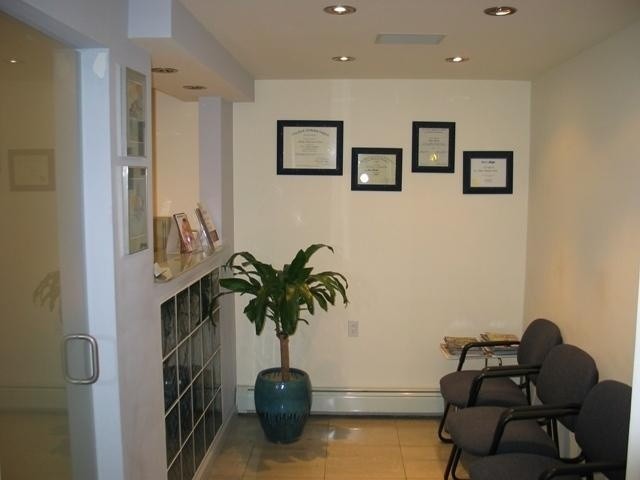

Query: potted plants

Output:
[209,244,349,444]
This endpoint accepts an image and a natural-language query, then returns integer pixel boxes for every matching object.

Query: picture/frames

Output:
[173,213,199,252]
[277,121,513,194]
[194,206,222,251]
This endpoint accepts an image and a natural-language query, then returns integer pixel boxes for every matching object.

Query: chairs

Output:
[439,319,632,479]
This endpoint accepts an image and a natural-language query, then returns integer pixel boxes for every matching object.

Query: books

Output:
[440,328,521,359]
